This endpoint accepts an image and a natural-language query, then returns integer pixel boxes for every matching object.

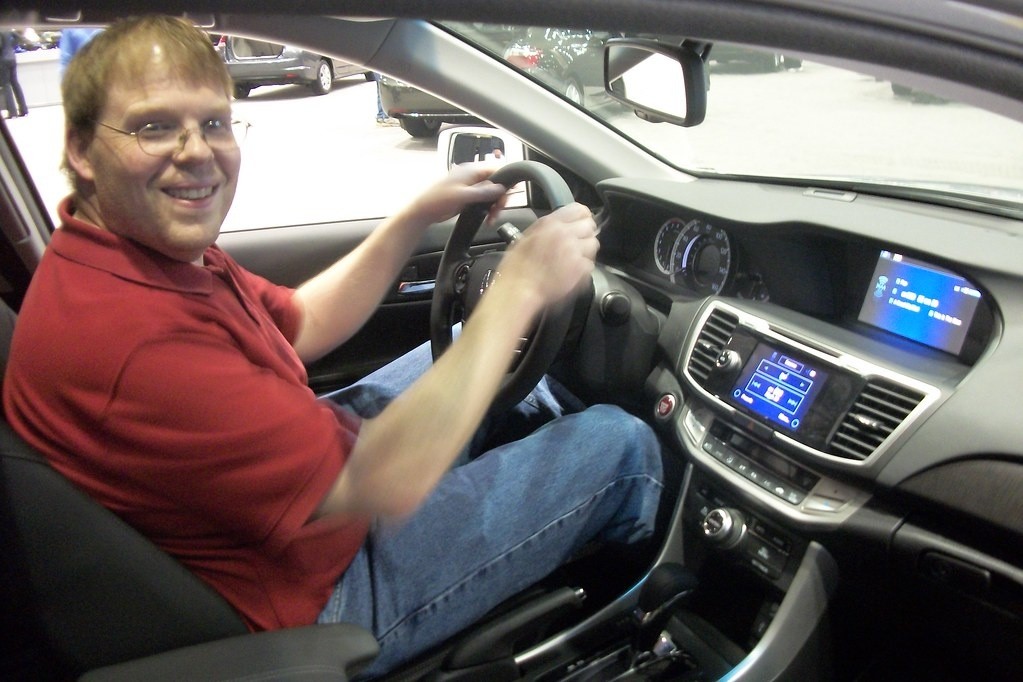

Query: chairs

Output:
[0,304,569,682]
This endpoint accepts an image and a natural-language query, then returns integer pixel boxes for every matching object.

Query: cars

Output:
[9,29,62,55]
[375,20,664,143]
[208,37,379,98]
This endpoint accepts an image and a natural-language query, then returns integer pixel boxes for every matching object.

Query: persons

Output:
[3,15,665,682]
[0,29,29,119]
[492,136,503,158]
[59,26,107,82]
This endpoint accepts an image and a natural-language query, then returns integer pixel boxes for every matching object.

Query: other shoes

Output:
[377,117,401,127]
[4,111,18,119]
[17,111,28,117]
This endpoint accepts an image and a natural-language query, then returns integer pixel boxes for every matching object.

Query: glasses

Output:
[86,113,251,158]
